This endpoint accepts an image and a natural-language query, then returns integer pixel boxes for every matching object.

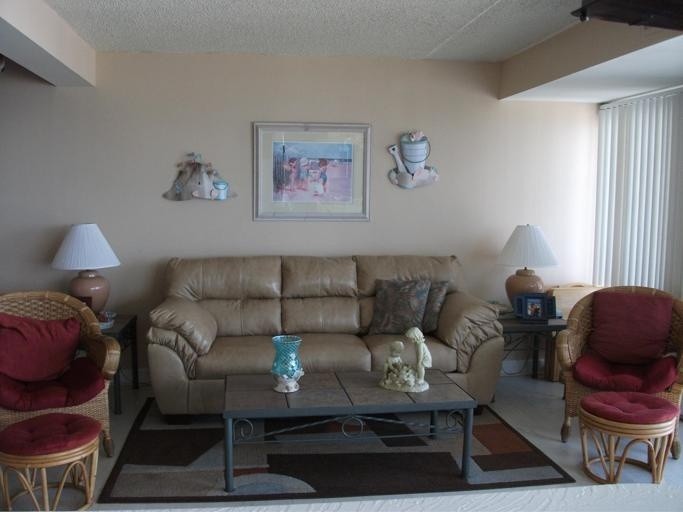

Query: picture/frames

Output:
[252,121,371,221]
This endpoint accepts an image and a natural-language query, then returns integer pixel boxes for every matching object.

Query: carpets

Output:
[97,397,576,504]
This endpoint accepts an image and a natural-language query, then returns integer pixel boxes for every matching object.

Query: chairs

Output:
[0,291,119,460]
[560,284,683,460]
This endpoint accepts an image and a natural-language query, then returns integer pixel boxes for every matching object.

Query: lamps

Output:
[52,222,121,321]
[497,224,560,309]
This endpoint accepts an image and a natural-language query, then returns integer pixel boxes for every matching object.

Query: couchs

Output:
[146,254,501,408]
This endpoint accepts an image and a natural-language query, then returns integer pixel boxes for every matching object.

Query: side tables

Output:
[103,315,139,417]
[498,313,567,380]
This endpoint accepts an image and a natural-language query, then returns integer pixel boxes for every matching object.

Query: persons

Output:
[406,327,432,385]
[529,302,540,317]
[384,341,404,379]
[283,157,328,196]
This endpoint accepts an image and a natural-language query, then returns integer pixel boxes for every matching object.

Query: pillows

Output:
[423,280,449,334]
[1,312,80,381]
[369,278,430,336]
[590,290,673,364]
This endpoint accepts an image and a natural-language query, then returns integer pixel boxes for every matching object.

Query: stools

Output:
[1,413,103,511]
[577,392,681,485]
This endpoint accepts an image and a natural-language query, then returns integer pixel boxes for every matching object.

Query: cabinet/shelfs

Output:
[545,284,601,318]
